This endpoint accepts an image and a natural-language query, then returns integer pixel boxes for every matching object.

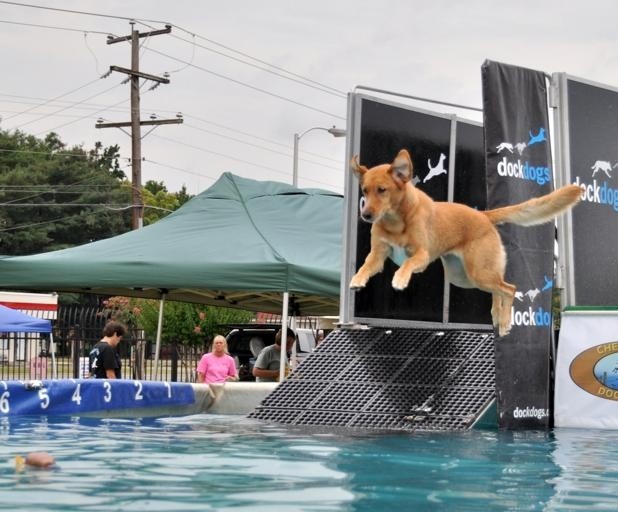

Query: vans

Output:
[209,322,332,384]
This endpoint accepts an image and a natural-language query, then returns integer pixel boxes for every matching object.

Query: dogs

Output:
[348,149,586,339]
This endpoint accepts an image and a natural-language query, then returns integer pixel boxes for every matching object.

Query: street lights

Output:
[293,128,345,187]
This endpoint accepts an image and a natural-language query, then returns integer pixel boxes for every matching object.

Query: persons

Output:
[251,326,295,382]
[196,334,240,383]
[88,321,127,380]
